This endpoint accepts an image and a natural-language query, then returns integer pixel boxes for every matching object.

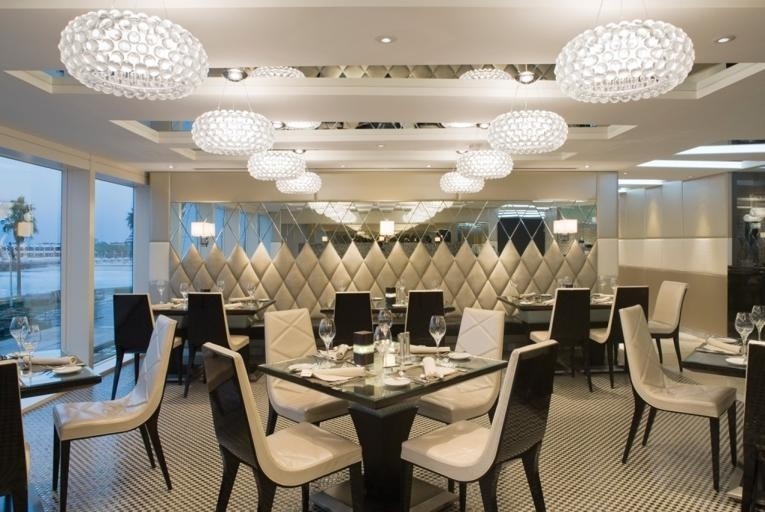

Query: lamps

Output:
[247,151,306,181]
[190,69,276,158]
[190,221,210,246]
[379,220,395,244]
[553,19,696,104]
[456,124,514,178]
[487,64,569,155]
[58,8,209,101]
[440,172,485,193]
[275,171,322,195]
[554,209,578,239]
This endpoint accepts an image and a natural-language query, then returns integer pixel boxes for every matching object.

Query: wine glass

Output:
[155,280,165,304]
[247,283,255,305]
[429,315,446,361]
[374,326,392,371]
[10,316,29,369]
[735,312,754,363]
[180,282,188,304]
[217,281,225,292]
[598,275,606,295]
[319,319,336,355]
[610,277,619,295]
[378,310,392,337]
[561,279,573,288]
[21,324,41,379]
[752,305,765,341]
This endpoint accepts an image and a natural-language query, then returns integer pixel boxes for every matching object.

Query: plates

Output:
[448,352,470,360]
[51,366,82,375]
[383,376,411,388]
[725,356,747,366]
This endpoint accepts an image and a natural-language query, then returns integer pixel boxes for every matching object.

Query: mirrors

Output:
[168,202,598,261]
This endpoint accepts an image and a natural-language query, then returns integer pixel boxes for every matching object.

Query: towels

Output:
[409,345,451,354]
[229,296,257,302]
[315,365,367,375]
[592,297,611,304]
[22,355,76,366]
[225,303,243,309]
[422,356,438,380]
[704,338,742,355]
[335,343,348,360]
[542,298,556,306]
[519,292,536,299]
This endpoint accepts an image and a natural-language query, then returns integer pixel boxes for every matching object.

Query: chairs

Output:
[416,307,506,493]
[529,289,593,393]
[729,341,765,512]
[404,290,445,348]
[183,293,250,400]
[648,281,688,372]
[589,287,649,389]
[53,314,179,511]
[400,340,560,512]
[618,304,737,491]
[675,371,681,373]
[110,293,183,400]
[0,360,29,512]
[333,291,373,346]
[264,307,350,437]
[201,341,362,512]
